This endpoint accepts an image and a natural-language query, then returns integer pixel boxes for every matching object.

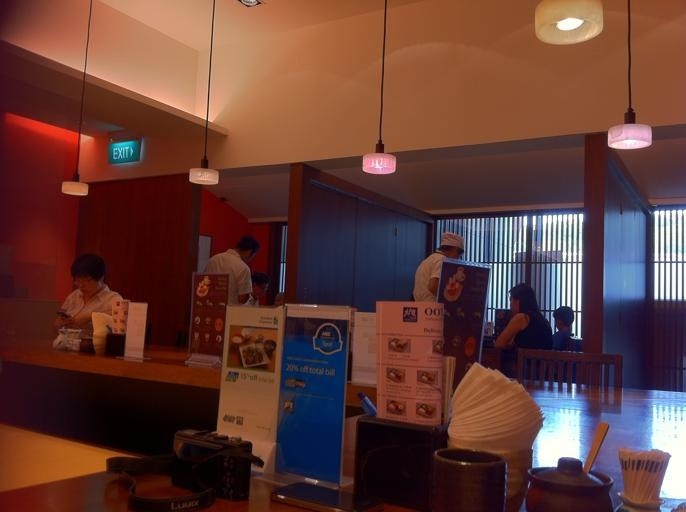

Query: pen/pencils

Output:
[355,391,376,418]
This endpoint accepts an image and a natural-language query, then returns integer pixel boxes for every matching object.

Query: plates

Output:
[238,344,271,368]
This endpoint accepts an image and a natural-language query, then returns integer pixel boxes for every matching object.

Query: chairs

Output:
[515,346,623,389]
[548,336,584,384]
[520,379,624,416]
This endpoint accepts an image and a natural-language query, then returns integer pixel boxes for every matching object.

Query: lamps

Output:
[534,1,604,47]
[60,0,93,198]
[189,1,220,186]
[361,1,399,176]
[606,0,654,154]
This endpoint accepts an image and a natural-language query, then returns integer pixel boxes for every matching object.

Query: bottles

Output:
[524,457,615,512]
[616,492,665,512]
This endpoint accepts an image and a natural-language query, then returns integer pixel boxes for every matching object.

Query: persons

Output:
[494,283,553,349]
[413,232,464,301]
[56,252,122,330]
[203,235,261,305]
[552,306,574,349]
[246,271,272,306]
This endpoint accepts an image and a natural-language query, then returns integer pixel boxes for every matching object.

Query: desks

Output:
[515,376,685,511]
[483,335,516,373]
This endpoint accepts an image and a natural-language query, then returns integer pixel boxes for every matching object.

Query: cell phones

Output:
[271,482,384,512]
[56,311,71,319]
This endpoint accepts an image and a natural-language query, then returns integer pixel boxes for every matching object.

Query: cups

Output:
[431,448,507,512]
[80,332,126,356]
[447,441,533,512]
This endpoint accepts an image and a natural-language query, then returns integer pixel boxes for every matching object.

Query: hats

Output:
[439,231,465,251]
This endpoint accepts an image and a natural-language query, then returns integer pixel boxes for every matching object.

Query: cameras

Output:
[171,429,250,501]
